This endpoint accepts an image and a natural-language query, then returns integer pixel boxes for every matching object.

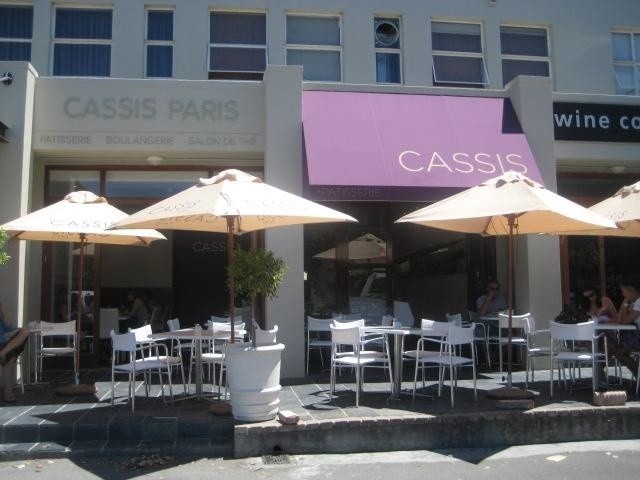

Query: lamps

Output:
[609,167,625,174]
[148,157,163,166]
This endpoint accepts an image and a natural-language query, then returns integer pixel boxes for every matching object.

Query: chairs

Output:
[15,352,24,395]
[41,320,76,382]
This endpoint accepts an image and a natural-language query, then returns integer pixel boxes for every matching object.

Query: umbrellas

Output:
[103,168,360,343]
[538,215,640,240]
[588,181,640,226]
[393,169,624,389]
[311,234,399,276]
[0,190,168,384]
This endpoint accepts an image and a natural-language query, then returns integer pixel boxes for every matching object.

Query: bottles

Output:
[393,318,398,328]
[195,323,199,332]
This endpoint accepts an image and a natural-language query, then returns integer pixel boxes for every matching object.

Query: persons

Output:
[0,305,31,402]
[578,287,619,386]
[108,292,151,363]
[475,281,520,371]
[613,278,640,394]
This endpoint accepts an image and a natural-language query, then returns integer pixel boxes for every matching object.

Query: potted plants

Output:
[222,241,286,422]
